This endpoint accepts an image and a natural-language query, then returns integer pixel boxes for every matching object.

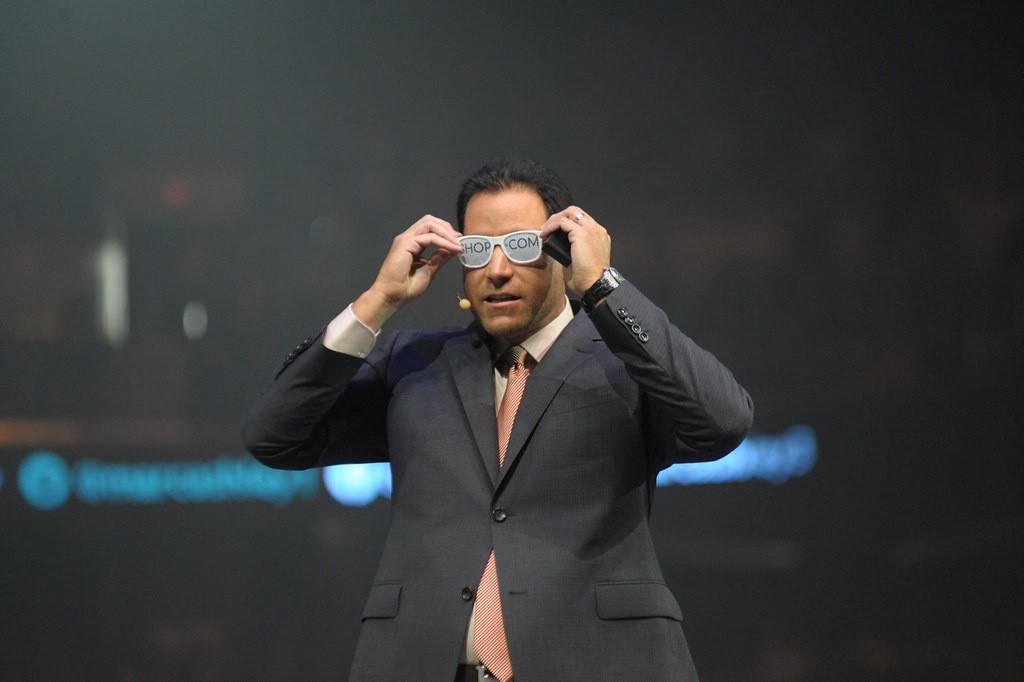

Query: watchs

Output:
[582,263,627,314]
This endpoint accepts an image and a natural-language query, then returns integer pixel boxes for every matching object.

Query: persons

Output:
[238,159,755,682]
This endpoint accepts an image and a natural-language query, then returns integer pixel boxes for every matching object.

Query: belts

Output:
[456,664,498,682]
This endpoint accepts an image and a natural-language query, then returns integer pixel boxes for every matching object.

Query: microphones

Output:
[456,293,471,310]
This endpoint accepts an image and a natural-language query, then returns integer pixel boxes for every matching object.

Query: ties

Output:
[474,346,531,682]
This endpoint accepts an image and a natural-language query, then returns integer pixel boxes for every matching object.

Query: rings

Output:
[573,212,585,223]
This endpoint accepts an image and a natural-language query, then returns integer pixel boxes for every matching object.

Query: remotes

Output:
[542,230,572,268]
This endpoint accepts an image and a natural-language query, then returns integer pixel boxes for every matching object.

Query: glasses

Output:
[457,230,544,268]
